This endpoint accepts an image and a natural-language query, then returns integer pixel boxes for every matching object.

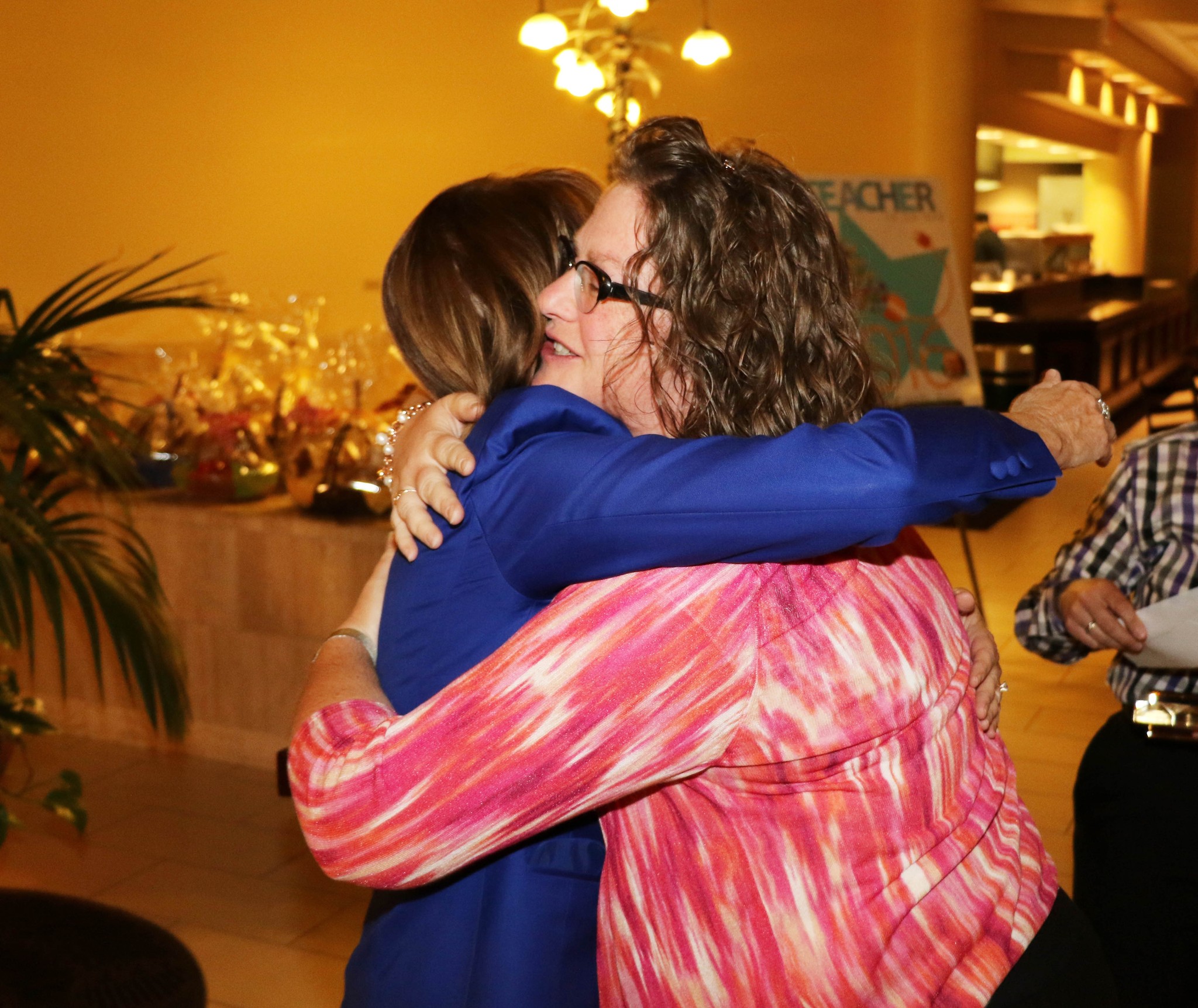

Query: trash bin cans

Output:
[0,886,207,1008]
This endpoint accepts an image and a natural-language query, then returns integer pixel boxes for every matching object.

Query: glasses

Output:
[556,235,672,312]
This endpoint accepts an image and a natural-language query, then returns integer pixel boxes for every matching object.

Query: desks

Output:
[17,493,394,768]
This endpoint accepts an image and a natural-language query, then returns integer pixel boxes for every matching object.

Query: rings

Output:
[393,489,416,500]
[1097,398,1110,420]
[998,681,1008,697]
[1085,620,1097,633]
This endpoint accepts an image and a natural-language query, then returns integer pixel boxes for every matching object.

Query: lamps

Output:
[517,0,731,144]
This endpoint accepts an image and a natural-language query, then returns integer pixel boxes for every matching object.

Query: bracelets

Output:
[310,628,377,666]
[375,401,432,492]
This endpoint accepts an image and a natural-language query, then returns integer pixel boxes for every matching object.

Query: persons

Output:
[973,212,1005,270]
[341,167,1116,1008]
[286,115,1113,1008]
[1015,420,1198,1008]
